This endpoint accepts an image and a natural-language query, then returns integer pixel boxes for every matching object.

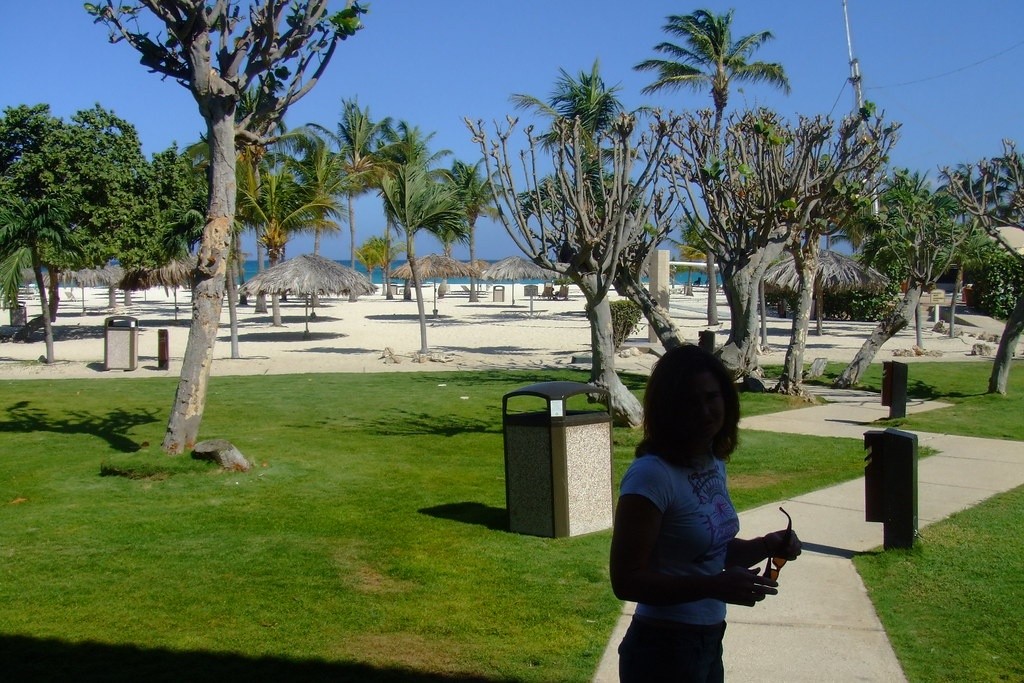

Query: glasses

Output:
[762,507,792,580]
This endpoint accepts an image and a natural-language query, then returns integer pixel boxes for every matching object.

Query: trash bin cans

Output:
[500,381,614,540]
[103,315,141,372]
[493,285,504,302]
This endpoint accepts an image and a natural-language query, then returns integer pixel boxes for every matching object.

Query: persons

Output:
[610,344,802,683]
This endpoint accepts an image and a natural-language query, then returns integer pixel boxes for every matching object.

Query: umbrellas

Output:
[238,254,379,337]
[467,256,545,304]
[19,253,198,320]
[764,249,891,334]
[389,253,480,317]
